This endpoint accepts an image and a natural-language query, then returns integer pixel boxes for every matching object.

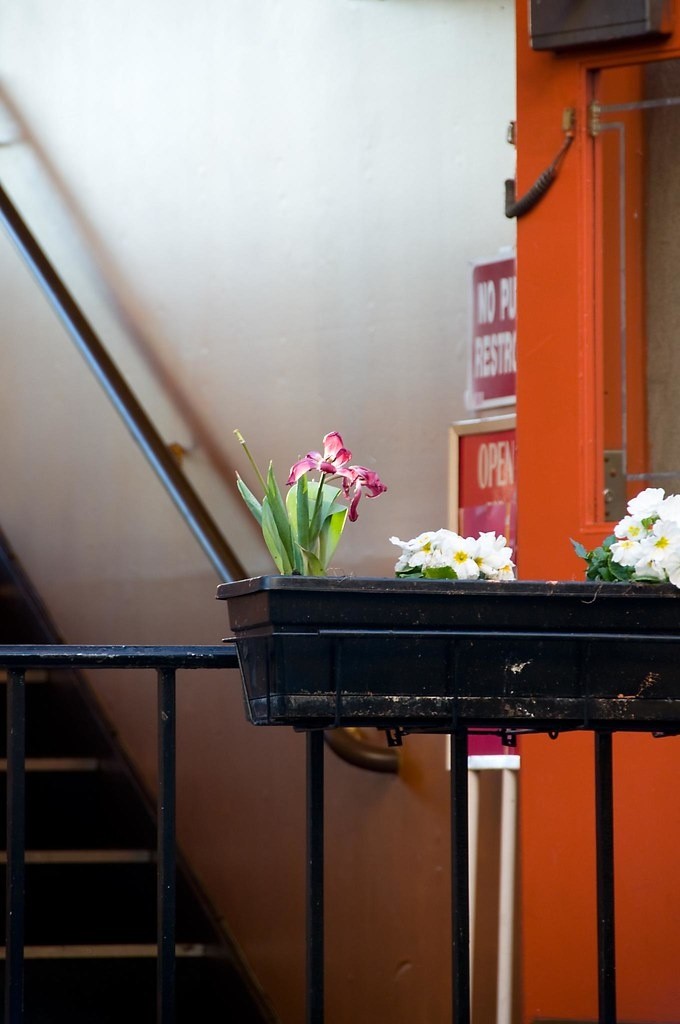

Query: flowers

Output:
[227,422,680,586]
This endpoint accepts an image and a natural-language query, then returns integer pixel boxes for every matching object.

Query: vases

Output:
[219,576,680,748]
